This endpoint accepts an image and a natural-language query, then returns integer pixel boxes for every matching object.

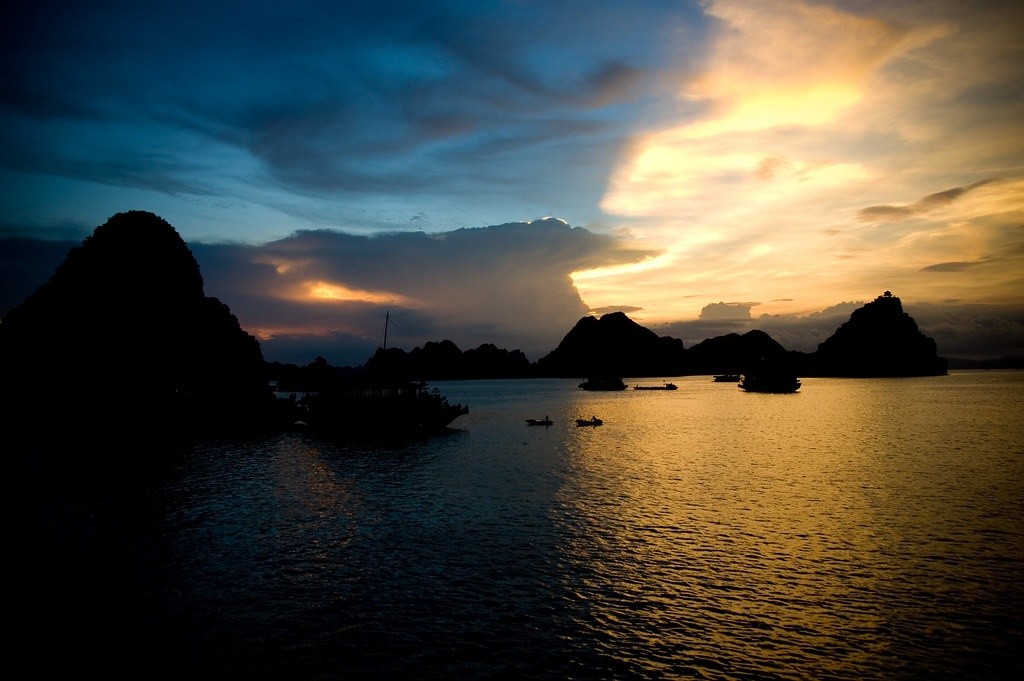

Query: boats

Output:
[713,374,742,382]
[634,383,678,390]
[524,419,553,425]
[577,364,629,392]
[294,307,470,440]
[738,355,802,393]
[576,419,603,427]
